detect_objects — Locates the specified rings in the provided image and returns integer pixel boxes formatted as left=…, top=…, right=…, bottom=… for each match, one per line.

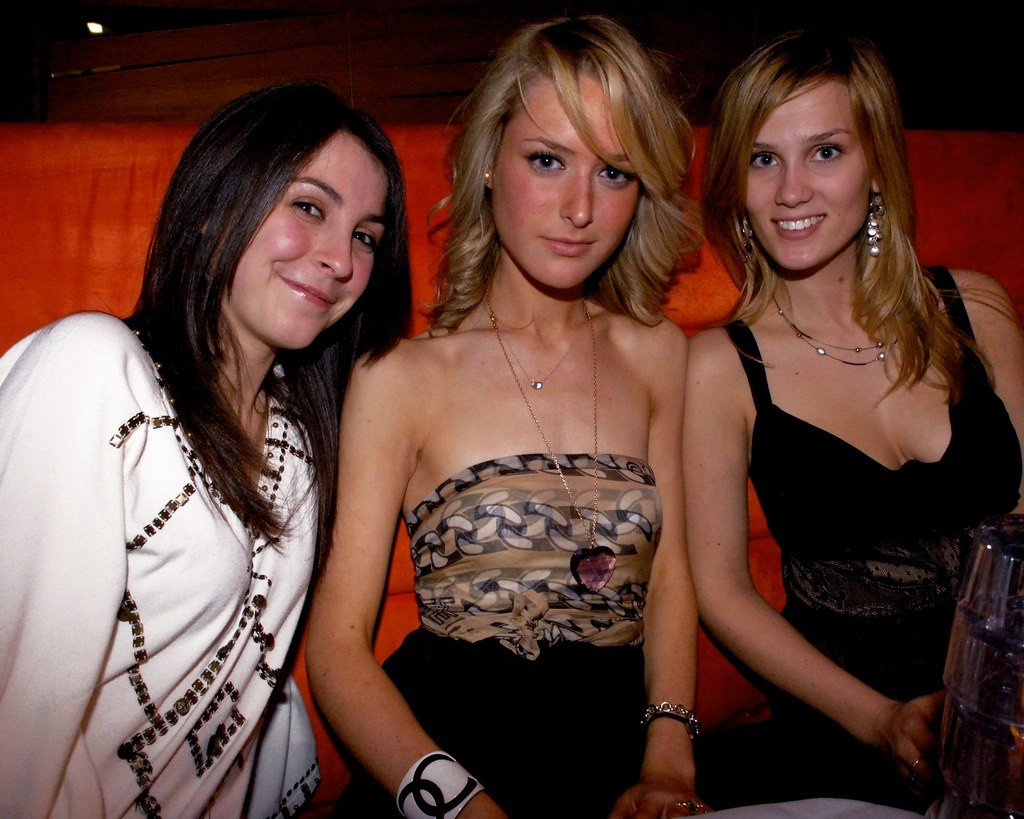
left=674, top=797, right=698, bottom=815
left=912, top=757, right=921, bottom=767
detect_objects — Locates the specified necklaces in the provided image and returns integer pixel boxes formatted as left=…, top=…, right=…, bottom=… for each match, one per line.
left=491, top=304, right=587, bottom=391
left=480, top=291, right=617, bottom=591
left=771, top=290, right=897, bottom=366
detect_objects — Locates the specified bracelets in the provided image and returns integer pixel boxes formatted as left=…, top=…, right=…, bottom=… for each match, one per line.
left=641, top=702, right=699, bottom=740
left=396, top=751, right=484, bottom=819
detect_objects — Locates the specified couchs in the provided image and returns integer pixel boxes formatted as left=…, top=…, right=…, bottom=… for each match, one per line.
left=3, top=117, right=1024, bottom=819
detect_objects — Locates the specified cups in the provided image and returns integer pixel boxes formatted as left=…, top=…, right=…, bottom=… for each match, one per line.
left=938, top=513, right=1024, bottom=819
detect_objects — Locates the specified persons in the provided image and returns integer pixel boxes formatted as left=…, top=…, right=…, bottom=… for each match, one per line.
left=0, top=83, right=411, bottom=819
left=309, top=13, right=690, bottom=819
left=684, top=27, right=1023, bottom=813
